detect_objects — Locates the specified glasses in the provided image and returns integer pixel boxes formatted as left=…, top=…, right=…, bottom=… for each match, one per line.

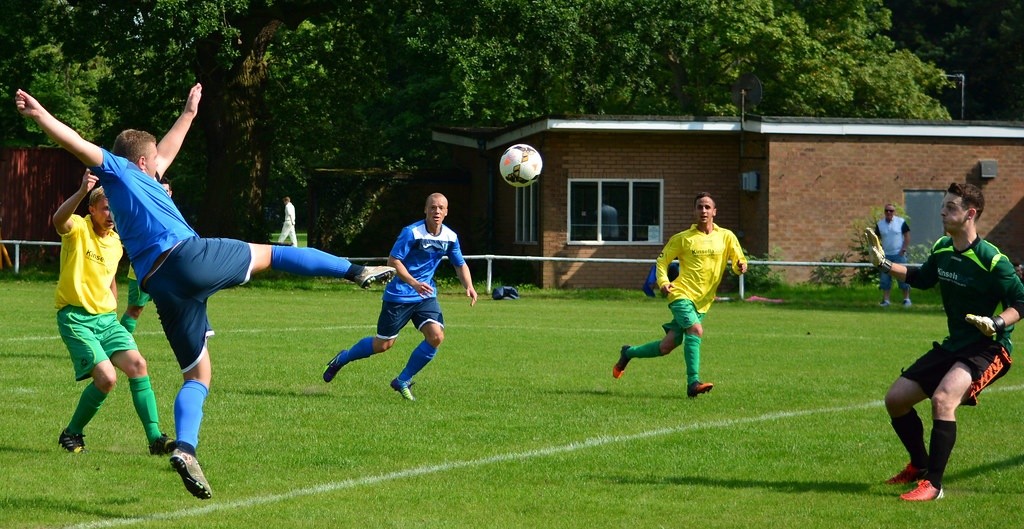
left=885, top=209, right=895, bottom=213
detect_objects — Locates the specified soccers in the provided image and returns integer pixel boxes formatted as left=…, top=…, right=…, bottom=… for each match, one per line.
left=500, top=143, right=542, bottom=187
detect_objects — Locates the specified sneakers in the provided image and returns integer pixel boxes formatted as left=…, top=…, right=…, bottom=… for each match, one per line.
left=688, top=381, right=713, bottom=398
left=880, top=300, right=890, bottom=306
left=887, top=462, right=930, bottom=484
left=391, top=377, right=414, bottom=401
left=323, top=350, right=346, bottom=382
left=150, top=436, right=178, bottom=454
left=900, top=479, right=945, bottom=502
left=356, top=266, right=397, bottom=289
left=170, top=449, right=212, bottom=499
left=59, top=428, right=84, bottom=453
left=613, top=346, right=630, bottom=379
left=902, top=299, right=911, bottom=306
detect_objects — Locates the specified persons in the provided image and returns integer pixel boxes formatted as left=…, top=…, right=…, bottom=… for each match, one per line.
left=16, top=82, right=396, bottom=500
left=875, top=206, right=911, bottom=306
left=120, top=177, right=172, bottom=335
left=278, top=197, right=297, bottom=247
left=323, top=193, right=478, bottom=400
left=864, top=183, right=1024, bottom=501
left=53, top=169, right=177, bottom=456
left=613, top=192, right=747, bottom=397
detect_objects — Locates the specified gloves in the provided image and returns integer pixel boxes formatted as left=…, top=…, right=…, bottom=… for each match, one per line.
left=863, top=227, right=884, bottom=267
left=965, top=313, right=996, bottom=336
